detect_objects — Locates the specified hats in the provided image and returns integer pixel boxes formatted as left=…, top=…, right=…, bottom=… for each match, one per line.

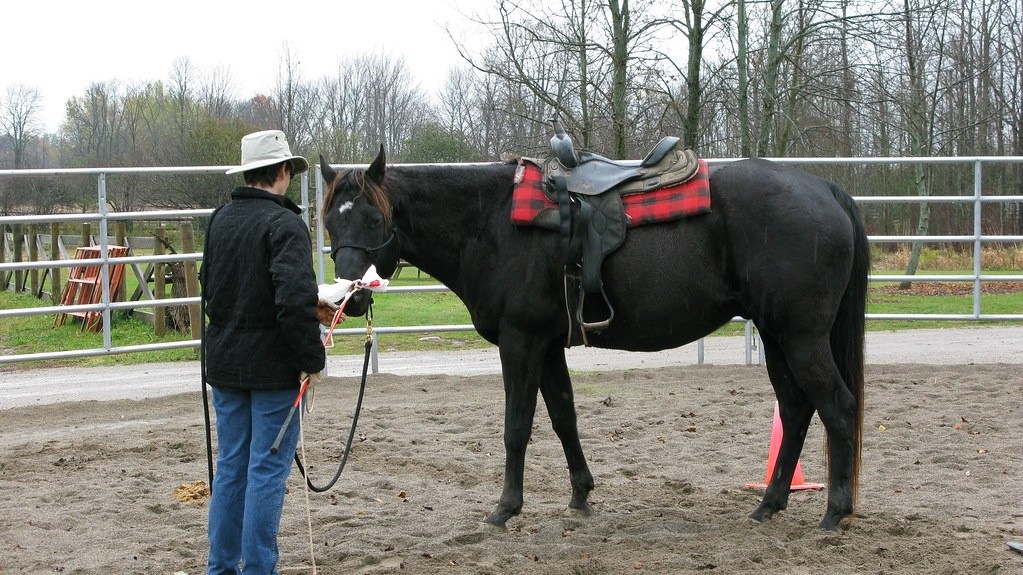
left=225, top=129, right=309, bottom=175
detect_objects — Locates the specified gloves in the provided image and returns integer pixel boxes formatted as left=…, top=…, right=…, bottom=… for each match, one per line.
left=299, top=371, right=322, bottom=389
left=316, top=297, right=346, bottom=326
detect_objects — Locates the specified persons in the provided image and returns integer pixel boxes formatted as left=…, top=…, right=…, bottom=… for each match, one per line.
left=200, top=130, right=345, bottom=575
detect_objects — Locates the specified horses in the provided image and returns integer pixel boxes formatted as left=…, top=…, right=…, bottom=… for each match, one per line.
left=319, top=143, right=871, bottom=534
